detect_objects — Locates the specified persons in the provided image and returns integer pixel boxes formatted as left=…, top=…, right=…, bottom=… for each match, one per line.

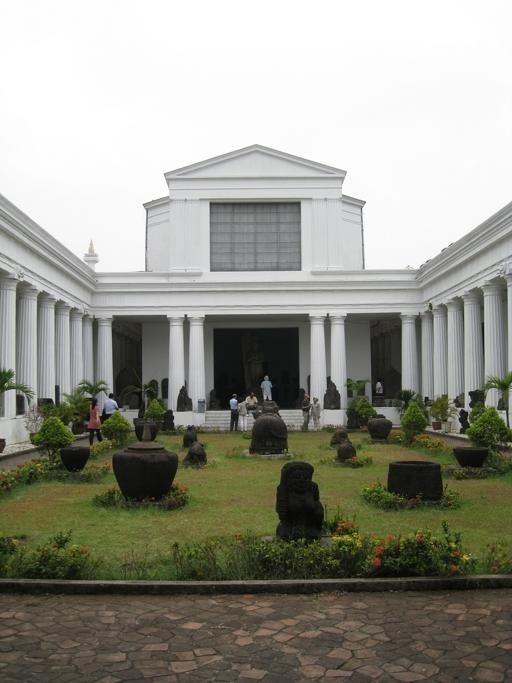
left=247, top=343, right=264, bottom=385
left=260, top=375, right=276, bottom=402
left=375, top=377, right=384, bottom=406
left=237, top=398, right=249, bottom=431
left=228, top=393, right=240, bottom=432
left=104, top=392, right=119, bottom=418
left=309, top=397, right=322, bottom=431
left=245, top=392, right=258, bottom=410
left=88, top=397, right=103, bottom=446
left=300, top=394, right=312, bottom=433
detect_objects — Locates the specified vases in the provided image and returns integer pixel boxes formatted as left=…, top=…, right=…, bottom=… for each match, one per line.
left=59, top=447, right=91, bottom=472
left=453, top=446, right=489, bottom=468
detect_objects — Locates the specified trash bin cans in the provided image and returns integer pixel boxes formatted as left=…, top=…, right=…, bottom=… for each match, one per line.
left=198, top=399, right=205, bottom=413
left=16, top=394, right=25, bottom=415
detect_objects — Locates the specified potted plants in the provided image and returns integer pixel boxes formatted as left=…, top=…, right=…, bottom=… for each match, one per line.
left=64, top=391, right=91, bottom=435
left=354, top=380, right=371, bottom=395
left=79, top=379, right=110, bottom=409
left=396, top=390, right=415, bottom=421
left=346, top=378, right=356, bottom=397
left=0, top=366, right=36, bottom=453
left=430, top=396, right=447, bottom=430
